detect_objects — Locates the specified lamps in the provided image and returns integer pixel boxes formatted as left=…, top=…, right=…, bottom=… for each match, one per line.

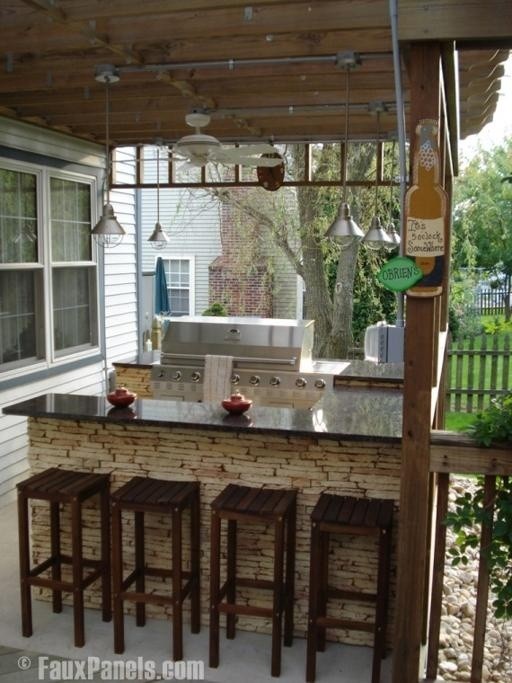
left=362, top=100, right=393, bottom=250
left=88, top=64, right=125, bottom=250
left=323, top=53, right=364, bottom=252
left=146, top=136, right=168, bottom=252
left=383, top=102, right=402, bottom=252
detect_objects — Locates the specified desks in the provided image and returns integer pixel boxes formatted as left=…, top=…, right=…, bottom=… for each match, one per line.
left=2, top=391, right=404, bottom=651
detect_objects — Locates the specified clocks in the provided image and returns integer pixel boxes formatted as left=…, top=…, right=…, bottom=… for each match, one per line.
left=256, top=149, right=287, bottom=191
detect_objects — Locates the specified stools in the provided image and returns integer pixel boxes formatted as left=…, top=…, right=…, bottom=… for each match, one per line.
left=14, top=466, right=114, bottom=648
left=305, top=491, right=396, bottom=682
left=109, top=475, right=202, bottom=661
left=208, top=481, right=299, bottom=677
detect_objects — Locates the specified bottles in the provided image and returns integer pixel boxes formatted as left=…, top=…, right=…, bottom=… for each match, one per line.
left=403, top=119, right=448, bottom=297
left=108, top=406, right=137, bottom=420
left=146, top=339, right=152, bottom=352
left=222, top=412, right=255, bottom=428
left=107, top=387, right=138, bottom=407
left=221, top=394, right=253, bottom=413
left=150, top=318, right=162, bottom=350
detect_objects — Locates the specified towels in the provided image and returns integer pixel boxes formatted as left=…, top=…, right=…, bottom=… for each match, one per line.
left=202, top=352, right=233, bottom=406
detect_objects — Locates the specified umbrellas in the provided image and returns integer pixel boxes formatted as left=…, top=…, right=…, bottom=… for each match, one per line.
left=154, top=257, right=170, bottom=317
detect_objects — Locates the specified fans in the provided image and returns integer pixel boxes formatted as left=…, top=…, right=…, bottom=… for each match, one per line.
left=108, top=109, right=284, bottom=177
